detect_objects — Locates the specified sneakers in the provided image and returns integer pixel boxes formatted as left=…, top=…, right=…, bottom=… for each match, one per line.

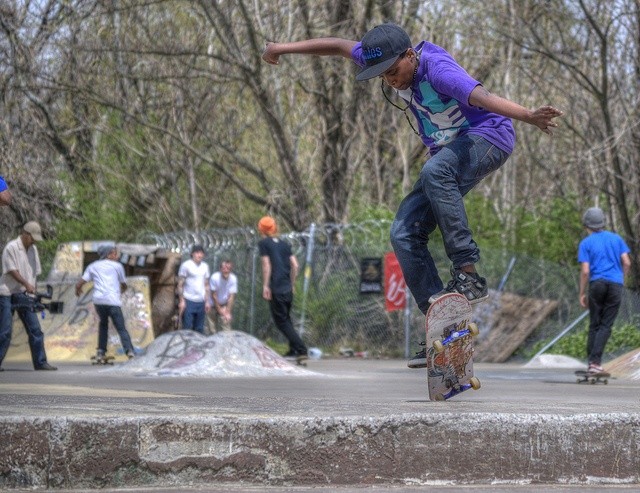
left=588, top=361, right=603, bottom=373
left=407, top=341, right=427, bottom=368
left=428, top=265, right=489, bottom=306
left=282, top=350, right=308, bottom=360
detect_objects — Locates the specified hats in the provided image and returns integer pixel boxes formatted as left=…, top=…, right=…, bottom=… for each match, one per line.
left=98, top=245, right=112, bottom=259
left=258, top=216, right=275, bottom=233
left=355, top=24, right=413, bottom=82
left=24, top=221, right=42, bottom=241
left=582, top=206, right=605, bottom=229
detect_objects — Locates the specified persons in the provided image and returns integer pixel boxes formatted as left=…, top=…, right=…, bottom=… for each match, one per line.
left=574, top=205, right=633, bottom=377
left=261, top=21, right=570, bottom=372
left=0, top=176, right=15, bottom=206
left=255, top=214, right=312, bottom=361
left=175, top=244, right=210, bottom=332
left=74, top=244, right=137, bottom=361
left=208, top=258, right=239, bottom=333
left=0, top=220, right=59, bottom=371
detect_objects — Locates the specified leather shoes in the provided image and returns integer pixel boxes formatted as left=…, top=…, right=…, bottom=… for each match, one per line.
left=35, top=363, right=57, bottom=370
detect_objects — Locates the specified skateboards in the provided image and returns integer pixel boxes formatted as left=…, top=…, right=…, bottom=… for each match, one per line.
left=574, top=370, right=611, bottom=384
left=283, top=356, right=309, bottom=367
left=425, top=294, right=480, bottom=401
left=92, top=356, right=116, bottom=365
